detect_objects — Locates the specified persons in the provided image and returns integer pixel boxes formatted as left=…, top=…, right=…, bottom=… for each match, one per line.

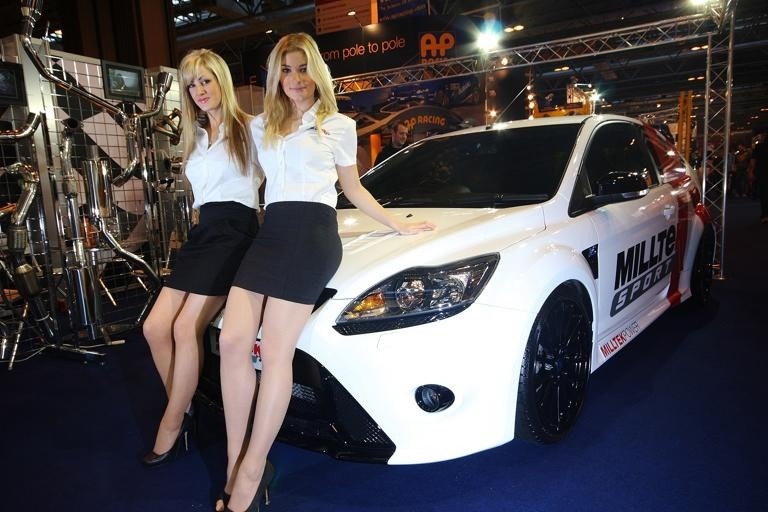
left=135, top=45, right=267, bottom=473
left=694, top=133, right=768, bottom=225
left=371, top=118, right=411, bottom=168
left=212, top=29, right=441, bottom=512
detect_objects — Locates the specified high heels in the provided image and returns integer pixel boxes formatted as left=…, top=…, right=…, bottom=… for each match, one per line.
left=223, top=458, right=276, bottom=512
left=141, top=411, right=192, bottom=466
left=214, top=488, right=231, bottom=512
left=187, top=399, right=200, bottom=436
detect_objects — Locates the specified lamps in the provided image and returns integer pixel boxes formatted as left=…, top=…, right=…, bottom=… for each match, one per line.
left=346, top=7, right=366, bottom=32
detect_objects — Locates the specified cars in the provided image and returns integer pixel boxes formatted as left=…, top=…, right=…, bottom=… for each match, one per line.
left=199, top=110, right=718, bottom=473
